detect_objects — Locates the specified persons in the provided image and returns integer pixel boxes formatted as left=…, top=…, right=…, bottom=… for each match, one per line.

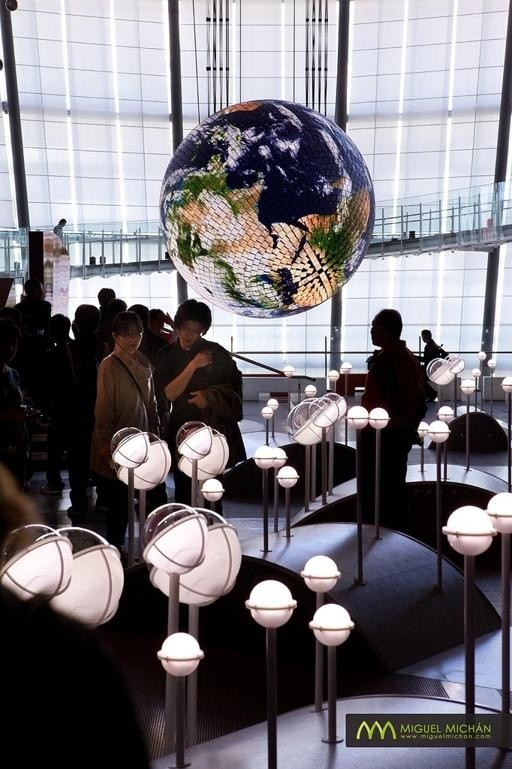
left=410, top=329, right=451, bottom=405
left=1, top=276, right=245, bottom=564
left=350, top=307, right=440, bottom=532
left=53, top=219, right=67, bottom=244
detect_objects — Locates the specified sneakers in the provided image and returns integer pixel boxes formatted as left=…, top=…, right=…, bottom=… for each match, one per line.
left=40, top=482, right=66, bottom=494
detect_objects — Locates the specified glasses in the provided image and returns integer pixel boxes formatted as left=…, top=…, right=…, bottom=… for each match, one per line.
left=117, top=331, right=144, bottom=339
left=180, top=326, right=203, bottom=335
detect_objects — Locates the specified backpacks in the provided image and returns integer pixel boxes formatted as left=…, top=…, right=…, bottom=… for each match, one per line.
left=438, top=344, right=450, bottom=360
